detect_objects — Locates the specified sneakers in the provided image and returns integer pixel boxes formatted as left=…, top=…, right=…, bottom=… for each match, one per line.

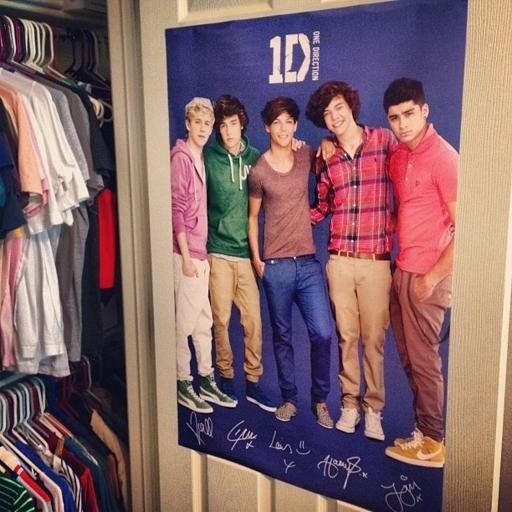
left=220, top=379, right=240, bottom=404
left=364, top=408, right=385, bottom=440
left=336, top=404, right=361, bottom=434
left=384, top=437, right=445, bottom=470
left=246, top=383, right=276, bottom=414
left=310, top=402, right=335, bottom=430
left=197, top=377, right=236, bottom=407
left=395, top=428, right=421, bottom=446
left=176, top=378, right=214, bottom=416
left=274, top=402, right=298, bottom=422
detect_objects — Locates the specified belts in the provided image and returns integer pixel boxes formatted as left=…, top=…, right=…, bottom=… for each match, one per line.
left=265, top=251, right=317, bottom=261
left=326, top=245, right=393, bottom=263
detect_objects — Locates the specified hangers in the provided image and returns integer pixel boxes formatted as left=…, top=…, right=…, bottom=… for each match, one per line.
left=0, top=13, right=116, bottom=130
left=0, top=341, right=130, bottom=482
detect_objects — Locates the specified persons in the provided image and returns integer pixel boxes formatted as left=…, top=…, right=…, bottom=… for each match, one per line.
left=383, top=75, right=458, bottom=468
left=304, top=79, right=401, bottom=443
left=202, top=93, right=307, bottom=415
left=246, top=96, right=334, bottom=430
left=170, top=95, right=237, bottom=414
